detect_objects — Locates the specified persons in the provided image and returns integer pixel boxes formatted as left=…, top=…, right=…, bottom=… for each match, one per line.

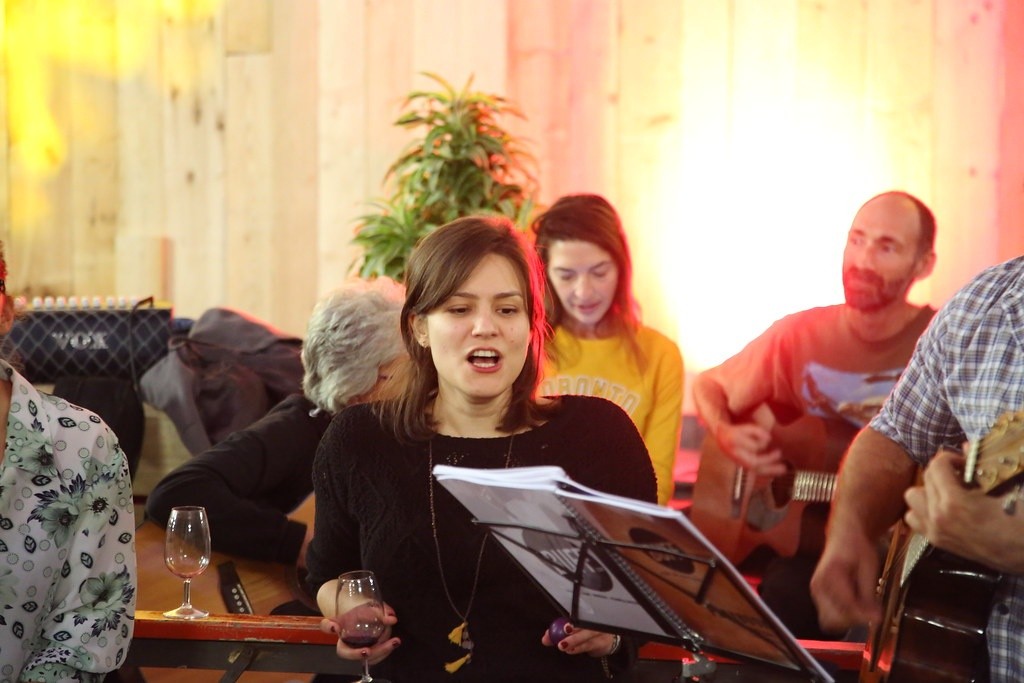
left=0, top=239, right=138, bottom=683
left=531, top=194, right=686, bottom=506
left=304, top=216, right=659, bottom=683
left=808, top=254, right=1024, bottom=683
left=145, top=275, right=421, bottom=569
left=691, top=189, right=945, bottom=644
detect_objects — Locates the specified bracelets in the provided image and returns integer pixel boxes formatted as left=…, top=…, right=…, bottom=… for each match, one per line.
left=600, top=634, right=622, bottom=680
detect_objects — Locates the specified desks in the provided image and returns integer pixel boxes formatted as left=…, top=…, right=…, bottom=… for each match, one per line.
left=126, top=520, right=365, bottom=683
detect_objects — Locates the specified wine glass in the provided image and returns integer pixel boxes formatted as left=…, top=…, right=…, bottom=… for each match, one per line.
left=162, top=505, right=212, bottom=620
left=335, top=569, right=387, bottom=683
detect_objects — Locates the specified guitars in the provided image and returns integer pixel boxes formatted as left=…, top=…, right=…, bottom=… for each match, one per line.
left=684, top=399, right=857, bottom=570
left=858, top=404, right=1024, bottom=683
